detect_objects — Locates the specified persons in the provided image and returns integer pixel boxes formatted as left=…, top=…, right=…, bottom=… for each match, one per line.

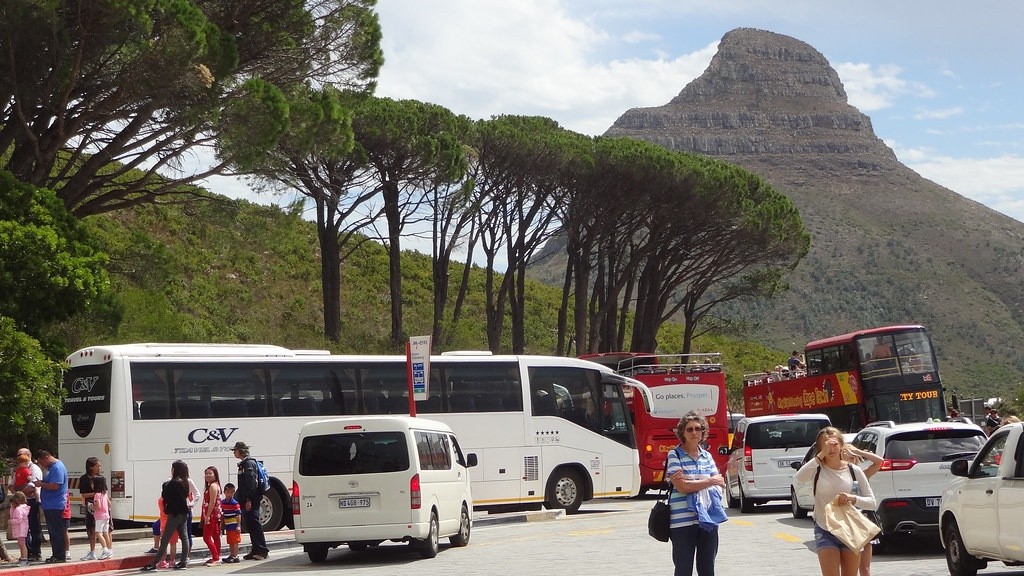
left=7, top=449, right=42, bottom=566
left=79, top=457, right=114, bottom=560
left=859, top=335, right=922, bottom=376
left=34, top=449, right=72, bottom=563
left=762, top=349, right=805, bottom=383
left=692, top=358, right=711, bottom=371
left=156, top=482, right=178, bottom=568
left=140, top=460, right=190, bottom=571
left=221, top=483, right=242, bottom=563
left=984, top=405, right=1002, bottom=437
left=144, top=478, right=200, bottom=562
left=201, top=466, right=223, bottom=567
left=231, top=441, right=269, bottom=560
left=667, top=409, right=727, bottom=576
left=841, top=443, right=883, bottom=576
left=0, top=486, right=14, bottom=562
left=947, top=406, right=959, bottom=418
left=796, top=426, right=876, bottom=576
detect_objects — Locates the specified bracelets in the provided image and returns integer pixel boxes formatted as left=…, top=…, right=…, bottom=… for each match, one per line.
left=816, top=456, right=822, bottom=462
left=247, top=499, right=250, bottom=501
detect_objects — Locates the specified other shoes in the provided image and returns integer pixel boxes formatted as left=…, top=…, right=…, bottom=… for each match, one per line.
left=98, top=549, right=113, bottom=560
left=243, top=552, right=255, bottom=560
left=205, top=558, right=222, bottom=566
left=166, top=559, right=175, bottom=567
left=0, top=554, right=42, bottom=567
left=222, top=555, right=240, bottom=563
left=65, top=551, right=71, bottom=560
left=46, top=555, right=66, bottom=563
left=155, top=561, right=166, bottom=568
left=253, top=554, right=269, bottom=560
left=144, top=548, right=159, bottom=555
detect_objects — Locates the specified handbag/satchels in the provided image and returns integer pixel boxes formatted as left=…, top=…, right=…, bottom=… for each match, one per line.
left=648, top=502, right=670, bottom=543
left=861, top=510, right=884, bottom=541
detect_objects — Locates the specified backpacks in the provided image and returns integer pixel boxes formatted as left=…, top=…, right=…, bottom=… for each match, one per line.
left=255, top=461, right=271, bottom=491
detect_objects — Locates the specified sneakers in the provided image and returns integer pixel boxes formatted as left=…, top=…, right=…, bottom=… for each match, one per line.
left=80, top=552, right=97, bottom=560
left=140, top=563, right=157, bottom=571
left=174, top=562, right=187, bottom=570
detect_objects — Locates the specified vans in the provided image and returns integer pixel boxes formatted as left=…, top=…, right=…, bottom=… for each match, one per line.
left=291, top=415, right=479, bottom=564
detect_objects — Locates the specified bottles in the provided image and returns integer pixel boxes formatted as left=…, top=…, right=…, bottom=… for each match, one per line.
left=851, top=481, right=861, bottom=496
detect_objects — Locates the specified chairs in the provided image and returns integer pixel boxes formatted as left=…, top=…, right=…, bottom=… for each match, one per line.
left=747, top=371, right=804, bottom=387
left=534, top=389, right=558, bottom=416
left=134, top=395, right=522, bottom=419
left=625, top=366, right=721, bottom=376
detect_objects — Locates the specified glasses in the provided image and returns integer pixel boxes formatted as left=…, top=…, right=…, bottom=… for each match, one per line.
left=683, top=427, right=702, bottom=432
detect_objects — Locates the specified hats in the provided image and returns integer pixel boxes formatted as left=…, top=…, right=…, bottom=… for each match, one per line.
left=231, top=441, right=249, bottom=451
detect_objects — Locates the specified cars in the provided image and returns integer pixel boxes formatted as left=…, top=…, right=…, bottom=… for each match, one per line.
left=939, top=422, right=1024, bottom=576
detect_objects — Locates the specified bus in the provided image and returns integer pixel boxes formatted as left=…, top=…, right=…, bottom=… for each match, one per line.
left=58, top=343, right=745, bottom=537
left=741, top=324, right=948, bottom=433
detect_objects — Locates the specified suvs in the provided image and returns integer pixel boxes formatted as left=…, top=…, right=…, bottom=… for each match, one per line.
left=717, top=413, right=833, bottom=515
left=790, top=417, right=990, bottom=551
